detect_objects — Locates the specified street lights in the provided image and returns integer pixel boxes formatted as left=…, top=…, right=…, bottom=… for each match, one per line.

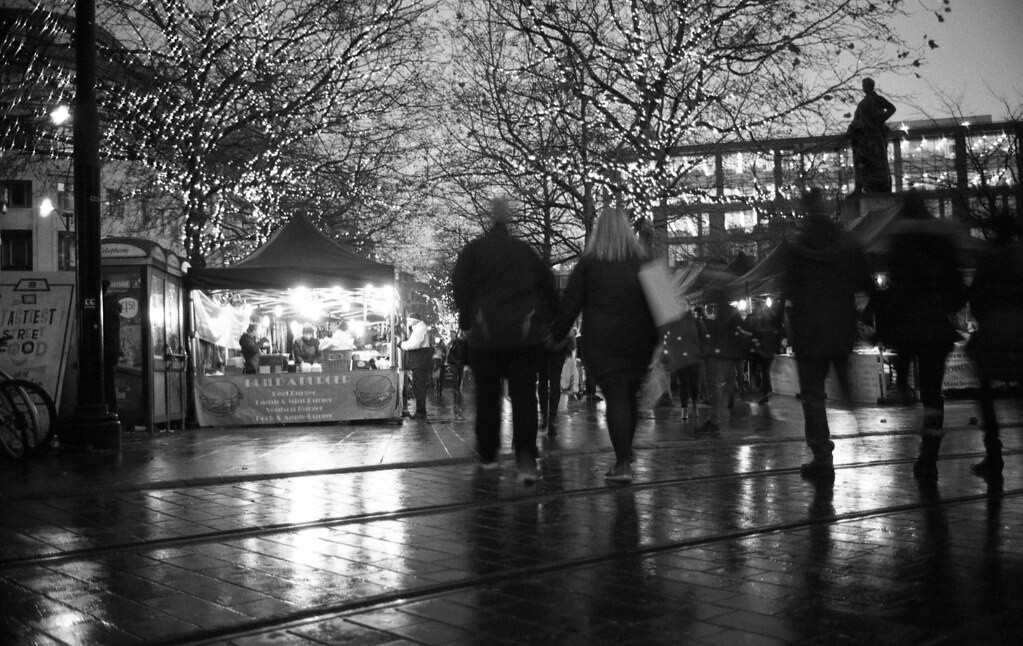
left=465, top=130, right=552, bottom=265
left=38, top=198, right=73, bottom=271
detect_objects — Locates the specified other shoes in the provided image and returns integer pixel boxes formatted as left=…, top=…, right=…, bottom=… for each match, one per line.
left=547, top=425, right=557, bottom=440
left=603, top=463, right=633, bottom=482
left=681, top=408, right=689, bottom=419
left=758, top=399, right=770, bottom=407
left=478, top=459, right=500, bottom=471
left=411, top=412, right=427, bottom=420
left=760, top=390, right=772, bottom=399
left=519, top=463, right=544, bottom=482
left=694, top=420, right=722, bottom=435
left=911, top=462, right=938, bottom=491
left=800, top=462, right=834, bottom=475
left=538, top=418, right=547, bottom=432
left=692, top=407, right=699, bottom=418
left=969, top=457, right=1006, bottom=473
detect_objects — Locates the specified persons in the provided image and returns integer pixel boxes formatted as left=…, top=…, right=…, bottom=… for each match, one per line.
left=403, top=312, right=468, bottom=419
left=240, top=311, right=320, bottom=374
left=451, top=201, right=554, bottom=484
left=966, top=211, right=1023, bottom=475
left=769, top=190, right=879, bottom=482
left=554, top=203, right=661, bottom=482
left=872, top=195, right=967, bottom=500
left=834, top=77, right=895, bottom=193
left=674, top=301, right=784, bottom=435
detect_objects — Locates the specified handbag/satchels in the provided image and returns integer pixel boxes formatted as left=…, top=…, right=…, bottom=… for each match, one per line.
left=636, top=261, right=685, bottom=329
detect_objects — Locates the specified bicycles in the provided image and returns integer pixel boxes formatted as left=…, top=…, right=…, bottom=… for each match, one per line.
left=0, top=368, right=41, bottom=462
left=0, top=379, right=57, bottom=456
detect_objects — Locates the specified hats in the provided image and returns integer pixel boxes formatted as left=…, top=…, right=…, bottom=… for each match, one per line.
left=407, top=313, right=421, bottom=321
left=302, top=326, right=315, bottom=333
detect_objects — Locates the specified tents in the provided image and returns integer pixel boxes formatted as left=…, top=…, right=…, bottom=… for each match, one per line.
left=672, top=239, right=795, bottom=300
left=182, top=212, right=395, bottom=431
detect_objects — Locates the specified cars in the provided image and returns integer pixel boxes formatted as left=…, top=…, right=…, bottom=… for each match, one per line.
left=408, top=345, right=460, bottom=395
